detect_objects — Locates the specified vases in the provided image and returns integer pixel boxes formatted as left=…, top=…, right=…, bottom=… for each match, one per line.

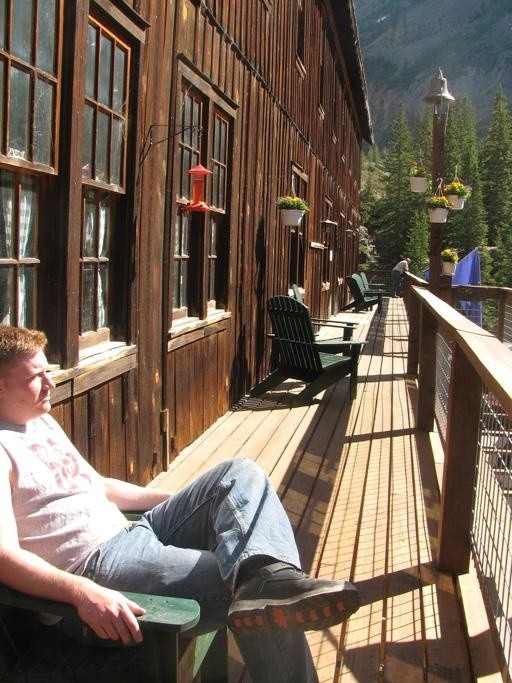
left=441, top=262, right=456, bottom=275
left=280, top=209, right=305, bottom=226
left=428, top=209, right=449, bottom=224
left=447, top=195, right=465, bottom=211
left=411, top=178, right=429, bottom=192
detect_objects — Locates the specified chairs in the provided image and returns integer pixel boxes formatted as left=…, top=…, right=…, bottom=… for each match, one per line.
left=251, top=295, right=370, bottom=406
left=293, top=284, right=359, bottom=354
left=0, top=511, right=227, bottom=683
left=345, top=272, right=392, bottom=314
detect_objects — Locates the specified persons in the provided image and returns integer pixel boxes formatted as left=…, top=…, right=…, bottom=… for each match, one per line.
left=391, top=257, right=411, bottom=298
left=0, top=323, right=366, bottom=681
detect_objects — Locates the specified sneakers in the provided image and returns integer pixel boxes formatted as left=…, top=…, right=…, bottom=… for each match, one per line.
left=226, top=561, right=362, bottom=638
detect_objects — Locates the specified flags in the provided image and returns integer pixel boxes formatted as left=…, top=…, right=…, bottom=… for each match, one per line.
left=452, top=247, right=482, bottom=329
left=424, top=268, right=430, bottom=282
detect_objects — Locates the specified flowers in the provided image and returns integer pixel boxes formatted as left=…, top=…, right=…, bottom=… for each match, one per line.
left=441, top=249, right=459, bottom=264
left=425, top=195, right=447, bottom=209
left=443, top=182, right=472, bottom=200
left=274, top=196, right=310, bottom=213
left=410, top=160, right=431, bottom=178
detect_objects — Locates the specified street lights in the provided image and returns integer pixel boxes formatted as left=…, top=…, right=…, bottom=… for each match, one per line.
left=423, top=66, right=455, bottom=289
